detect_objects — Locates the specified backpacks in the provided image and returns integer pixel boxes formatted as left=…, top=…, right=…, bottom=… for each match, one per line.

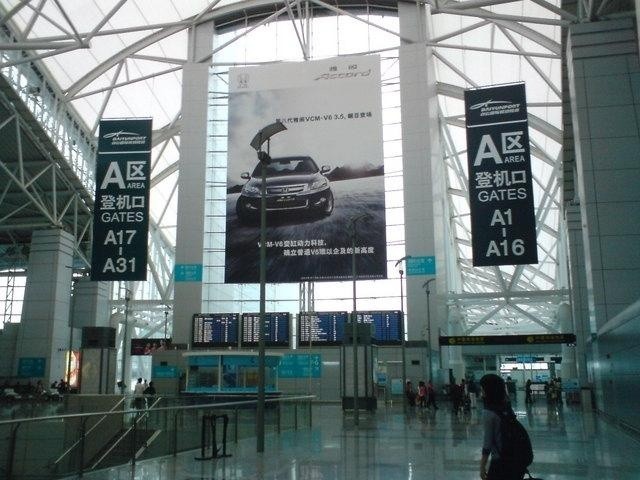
left=486, top=402, right=534, bottom=472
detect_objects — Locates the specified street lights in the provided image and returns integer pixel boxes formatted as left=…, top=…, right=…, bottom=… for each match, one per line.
left=164, top=310, right=169, bottom=339
left=118, top=286, right=132, bottom=395
left=346, top=214, right=441, bottom=434
left=64, top=266, right=87, bottom=392
left=252, top=122, right=288, bottom=461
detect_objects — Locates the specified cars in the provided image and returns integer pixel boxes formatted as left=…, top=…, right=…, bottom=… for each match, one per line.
left=237, top=154, right=335, bottom=225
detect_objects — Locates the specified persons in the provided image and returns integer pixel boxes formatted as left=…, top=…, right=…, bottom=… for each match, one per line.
left=0, top=379, right=69, bottom=398
left=404, top=409, right=567, bottom=446
left=479, top=374, right=533, bottom=480
left=134, top=378, right=155, bottom=409
left=405, top=376, right=563, bottom=409
